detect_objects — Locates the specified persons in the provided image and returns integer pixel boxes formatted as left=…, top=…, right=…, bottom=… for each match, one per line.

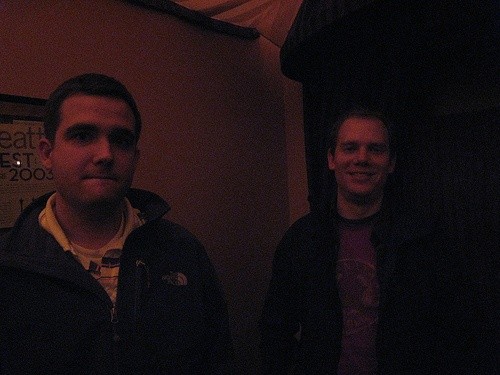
left=259, top=105, right=500, bottom=375
left=0, top=73, right=233, bottom=375
